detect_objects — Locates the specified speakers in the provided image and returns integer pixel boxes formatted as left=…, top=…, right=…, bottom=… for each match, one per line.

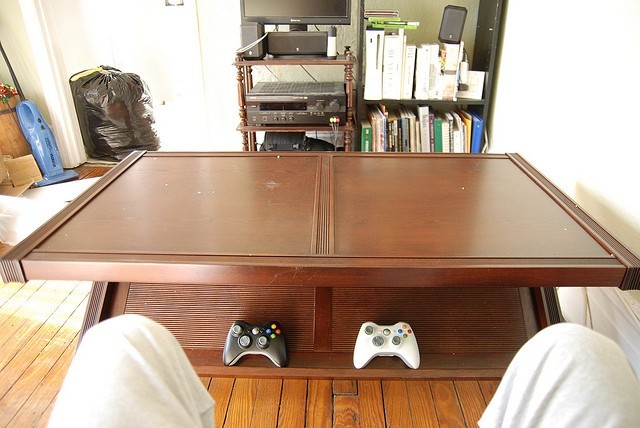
left=240, top=21, right=266, bottom=60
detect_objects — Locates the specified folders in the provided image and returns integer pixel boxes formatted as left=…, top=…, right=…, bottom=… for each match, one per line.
left=464, top=109, right=484, bottom=153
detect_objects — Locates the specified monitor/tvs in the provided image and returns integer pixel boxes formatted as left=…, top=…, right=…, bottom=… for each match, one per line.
left=240, top=0, right=351, bottom=31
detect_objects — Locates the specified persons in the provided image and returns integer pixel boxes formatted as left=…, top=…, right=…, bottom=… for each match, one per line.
left=42, top=313, right=640, bottom=428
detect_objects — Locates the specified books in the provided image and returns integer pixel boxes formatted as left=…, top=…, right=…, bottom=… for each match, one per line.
left=360, top=25, right=484, bottom=152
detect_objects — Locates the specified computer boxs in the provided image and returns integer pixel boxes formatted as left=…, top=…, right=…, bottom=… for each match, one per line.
left=266, top=30, right=327, bottom=55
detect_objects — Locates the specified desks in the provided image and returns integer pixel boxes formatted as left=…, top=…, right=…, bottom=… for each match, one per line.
left=0, top=151, right=640, bottom=379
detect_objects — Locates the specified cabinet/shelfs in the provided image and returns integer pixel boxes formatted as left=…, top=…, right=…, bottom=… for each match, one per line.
left=233, top=52, right=354, bottom=151
left=355, top=1, right=503, bottom=156
